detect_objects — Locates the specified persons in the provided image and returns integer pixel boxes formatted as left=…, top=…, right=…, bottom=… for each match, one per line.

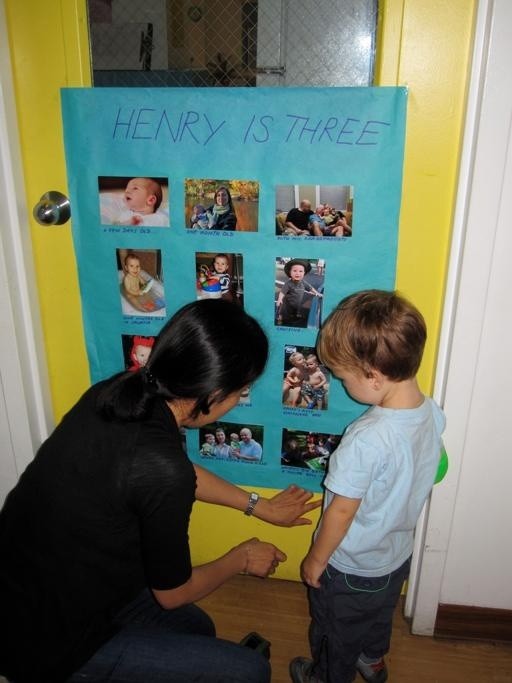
left=120, top=178, right=169, bottom=226
left=124, top=254, right=146, bottom=296
left=191, top=186, right=237, bottom=231
left=131, top=337, right=153, bottom=371
left=205, top=253, right=230, bottom=298
left=278, top=259, right=323, bottom=321
left=202, top=429, right=262, bottom=463
left=285, top=199, right=346, bottom=237
left=286, top=432, right=339, bottom=466
left=288, top=289, right=447, bottom=682
left=1, top=299, right=321, bottom=683
left=283, top=353, right=326, bottom=410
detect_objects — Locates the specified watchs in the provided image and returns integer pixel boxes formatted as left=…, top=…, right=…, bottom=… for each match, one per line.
left=245, top=492, right=259, bottom=516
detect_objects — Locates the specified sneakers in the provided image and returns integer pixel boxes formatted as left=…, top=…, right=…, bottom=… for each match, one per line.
left=290, top=656, right=355, bottom=683
left=354, top=651, right=388, bottom=683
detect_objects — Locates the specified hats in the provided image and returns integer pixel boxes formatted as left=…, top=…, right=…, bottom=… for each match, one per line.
left=281, top=259, right=315, bottom=278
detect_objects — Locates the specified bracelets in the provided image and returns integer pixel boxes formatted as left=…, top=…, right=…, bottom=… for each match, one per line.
left=236, top=547, right=249, bottom=577
